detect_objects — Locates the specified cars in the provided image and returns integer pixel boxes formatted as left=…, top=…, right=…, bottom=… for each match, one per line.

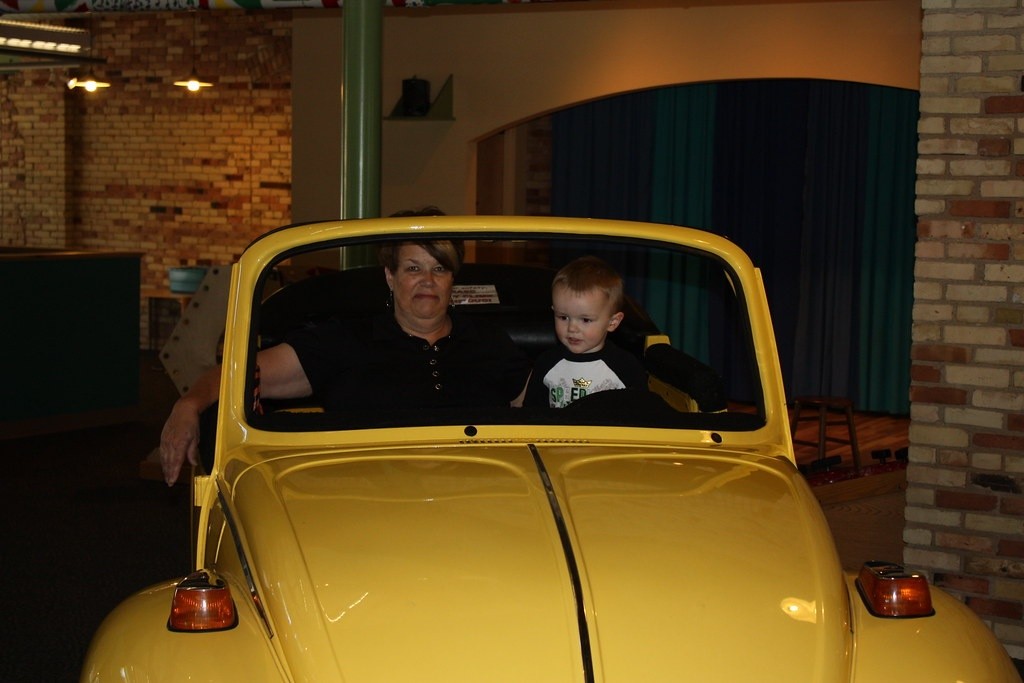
left=79, top=218, right=1024, bottom=683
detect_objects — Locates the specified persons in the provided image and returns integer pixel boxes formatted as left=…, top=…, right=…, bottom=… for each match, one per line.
left=519, top=257, right=661, bottom=397
left=160, top=212, right=533, bottom=488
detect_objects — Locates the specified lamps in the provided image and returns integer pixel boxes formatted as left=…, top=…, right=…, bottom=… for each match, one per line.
left=172, top=9, right=215, bottom=95
left=66, top=13, right=111, bottom=93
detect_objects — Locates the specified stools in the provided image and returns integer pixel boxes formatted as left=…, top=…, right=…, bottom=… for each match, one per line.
left=791, top=395, right=862, bottom=470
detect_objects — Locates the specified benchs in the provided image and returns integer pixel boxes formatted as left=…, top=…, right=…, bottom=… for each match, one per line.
left=257, top=262, right=647, bottom=432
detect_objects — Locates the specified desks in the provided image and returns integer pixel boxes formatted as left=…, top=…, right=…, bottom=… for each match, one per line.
left=142, top=287, right=194, bottom=351
